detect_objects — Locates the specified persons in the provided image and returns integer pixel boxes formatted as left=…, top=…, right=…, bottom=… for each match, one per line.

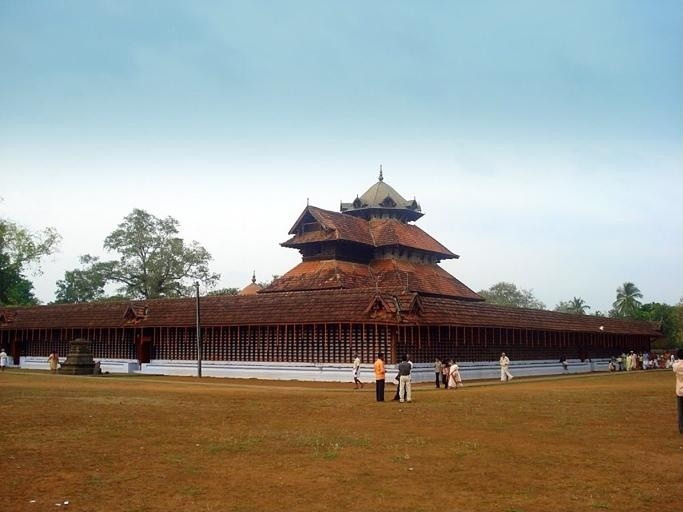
left=398, top=355, right=412, bottom=403
left=374, top=353, right=387, bottom=401
left=47, top=351, right=60, bottom=374
left=352, top=353, right=364, bottom=389
left=559, top=352, right=568, bottom=370
left=499, top=353, right=514, bottom=383
left=0, top=348, right=7, bottom=372
left=608, top=350, right=678, bottom=372
left=394, top=354, right=413, bottom=400
left=434, top=355, right=463, bottom=390
left=672, top=348, right=683, bottom=434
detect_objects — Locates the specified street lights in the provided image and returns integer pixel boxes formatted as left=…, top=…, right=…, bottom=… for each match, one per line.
left=193, top=282, right=201, bottom=378
